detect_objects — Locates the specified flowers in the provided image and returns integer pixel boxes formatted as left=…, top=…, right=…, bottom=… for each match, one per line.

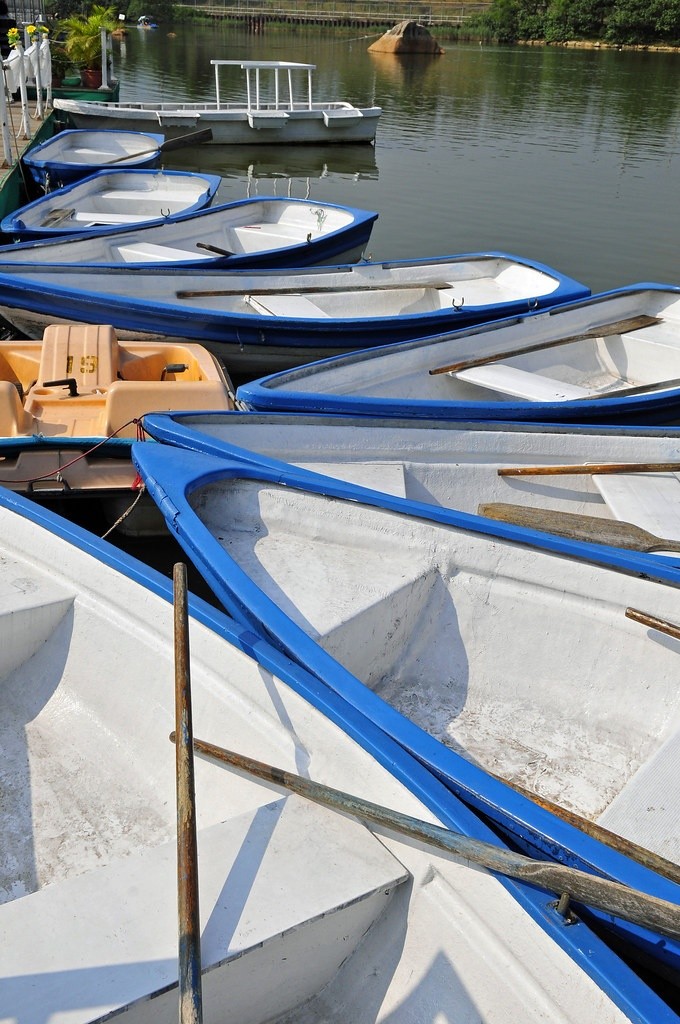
left=27, top=25, right=36, bottom=35
left=7, top=27, right=22, bottom=42
left=40, top=26, right=49, bottom=33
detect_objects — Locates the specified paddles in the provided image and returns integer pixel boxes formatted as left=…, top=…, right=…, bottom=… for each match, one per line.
left=425, top=311, right=668, bottom=380
left=99, top=125, right=216, bottom=166
left=471, top=495, right=680, bottom=571
left=567, top=372, right=680, bottom=404
left=163, top=556, right=213, bottom=1024
left=40, top=207, right=77, bottom=229
left=467, top=755, right=680, bottom=896
left=171, top=279, right=455, bottom=302
left=622, top=602, right=680, bottom=649
left=496, top=451, right=680, bottom=479
left=189, top=237, right=244, bottom=257
left=165, top=725, right=680, bottom=961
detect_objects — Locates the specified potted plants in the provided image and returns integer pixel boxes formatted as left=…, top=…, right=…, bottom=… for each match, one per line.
left=59, top=6, right=130, bottom=89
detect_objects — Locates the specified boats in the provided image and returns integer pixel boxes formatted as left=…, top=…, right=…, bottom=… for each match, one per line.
left=133, top=408, right=679, bottom=990
left=235, top=282, right=680, bottom=421
left=0, top=195, right=380, bottom=265
left=0, top=324, right=243, bottom=554
left=24, top=129, right=165, bottom=195
left=0, top=168, right=222, bottom=243
left=52, top=59, right=383, bottom=146
left=0, top=251, right=594, bottom=388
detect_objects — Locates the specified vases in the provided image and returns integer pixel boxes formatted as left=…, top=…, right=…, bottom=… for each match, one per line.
left=31, top=36, right=38, bottom=42
left=13, top=41, right=21, bottom=46
left=42, top=33, right=48, bottom=40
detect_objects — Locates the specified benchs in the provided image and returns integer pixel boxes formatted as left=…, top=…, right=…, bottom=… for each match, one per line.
left=64, top=212, right=163, bottom=227
left=92, top=188, right=200, bottom=215
left=590, top=310, right=680, bottom=392
left=444, top=362, right=601, bottom=402
left=244, top=292, right=331, bottom=320
left=62, top=145, right=150, bottom=164
left=0, top=789, right=413, bottom=1024
left=110, top=241, right=216, bottom=263
left=430, top=277, right=519, bottom=310
left=594, top=726, right=680, bottom=866
left=227, top=221, right=327, bottom=254
left=586, top=461, right=680, bottom=558
